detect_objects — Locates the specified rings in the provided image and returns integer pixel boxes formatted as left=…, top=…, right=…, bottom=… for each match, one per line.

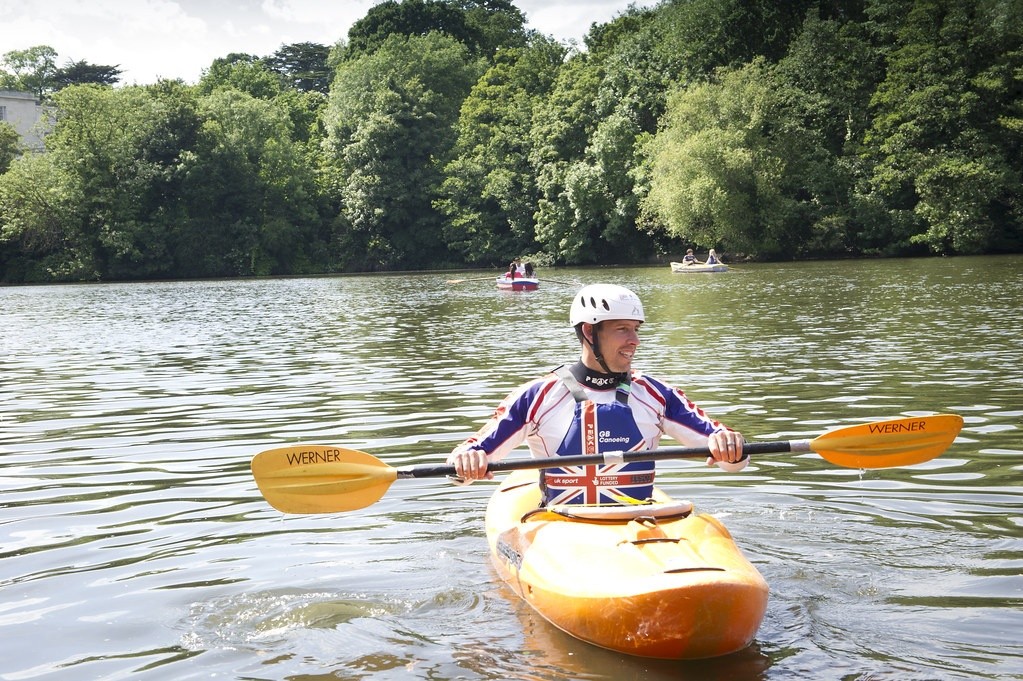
left=727, top=441, right=735, bottom=445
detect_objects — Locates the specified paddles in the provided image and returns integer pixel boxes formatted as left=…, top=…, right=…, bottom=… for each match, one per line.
left=446, top=277, right=497, bottom=284
left=252, top=413, right=963, bottom=514
left=537, top=277, right=566, bottom=284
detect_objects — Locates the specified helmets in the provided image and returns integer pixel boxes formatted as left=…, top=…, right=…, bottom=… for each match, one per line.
left=569, top=283, right=645, bottom=328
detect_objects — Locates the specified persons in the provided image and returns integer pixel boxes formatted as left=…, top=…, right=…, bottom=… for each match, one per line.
left=683, top=249, right=698, bottom=264
left=520, top=262, right=536, bottom=279
left=513, top=257, right=525, bottom=274
left=445, top=284, right=752, bottom=512
left=705, top=249, right=717, bottom=264
left=505, top=263, right=524, bottom=279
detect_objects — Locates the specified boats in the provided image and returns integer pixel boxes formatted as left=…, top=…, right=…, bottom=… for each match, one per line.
left=483, top=467, right=771, bottom=660
left=670, top=261, right=729, bottom=272
left=496, top=274, right=540, bottom=292
